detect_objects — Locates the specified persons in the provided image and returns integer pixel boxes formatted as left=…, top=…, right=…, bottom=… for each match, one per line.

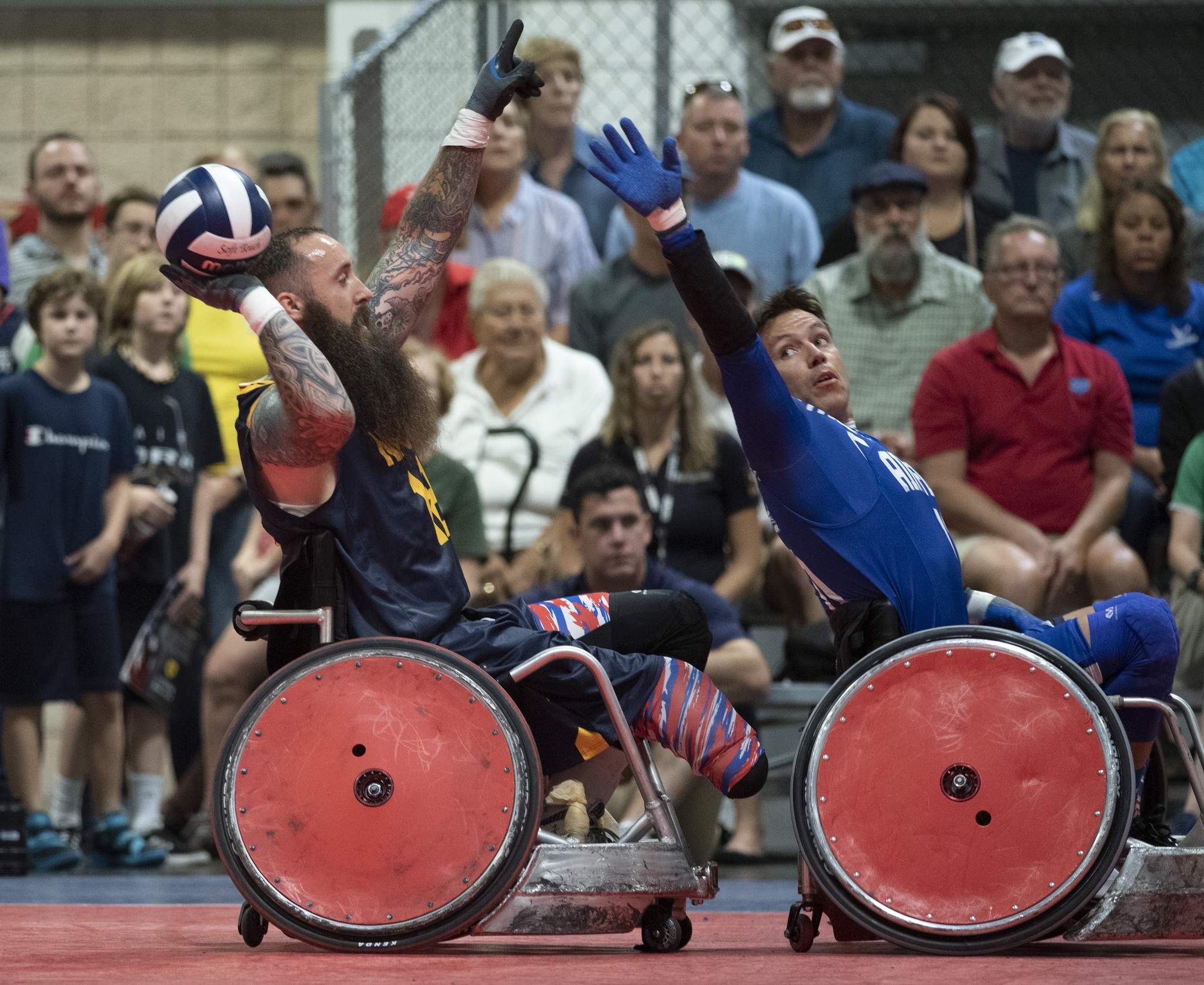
left=0, top=0, right=1204, bottom=874
left=575, top=116, right=1199, bottom=866
left=784, top=154, right=999, bottom=544
left=901, top=206, right=1151, bottom=612
left=152, top=20, right=766, bottom=800
left=522, top=317, right=762, bottom=622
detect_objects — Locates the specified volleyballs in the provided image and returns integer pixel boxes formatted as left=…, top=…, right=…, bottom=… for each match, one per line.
left=155, top=163, right=273, bottom=279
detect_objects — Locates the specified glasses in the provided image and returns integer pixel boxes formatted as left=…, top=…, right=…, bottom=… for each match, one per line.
left=858, top=191, right=923, bottom=212
left=998, top=259, right=1059, bottom=285
left=684, top=81, right=744, bottom=101
left=788, top=50, right=832, bottom=63
left=1017, top=67, right=1065, bottom=81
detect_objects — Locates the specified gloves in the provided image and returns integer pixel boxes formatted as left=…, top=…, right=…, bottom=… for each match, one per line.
left=159, top=263, right=264, bottom=314
left=466, top=19, right=545, bottom=122
left=588, top=117, right=688, bottom=232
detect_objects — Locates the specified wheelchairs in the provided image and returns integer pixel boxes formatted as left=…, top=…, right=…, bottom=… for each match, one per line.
left=208, top=599, right=717, bottom=954
left=784, top=622, right=1203, bottom=958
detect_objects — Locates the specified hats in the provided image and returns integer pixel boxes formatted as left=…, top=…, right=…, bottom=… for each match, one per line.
left=768, top=5, right=843, bottom=54
left=849, top=162, right=929, bottom=197
left=997, top=31, right=1079, bottom=72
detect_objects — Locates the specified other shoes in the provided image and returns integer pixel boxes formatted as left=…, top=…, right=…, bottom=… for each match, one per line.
left=166, top=814, right=220, bottom=863
left=1132, top=819, right=1178, bottom=847
left=25, top=811, right=79, bottom=876
left=81, top=806, right=165, bottom=870
left=720, top=837, right=777, bottom=864
